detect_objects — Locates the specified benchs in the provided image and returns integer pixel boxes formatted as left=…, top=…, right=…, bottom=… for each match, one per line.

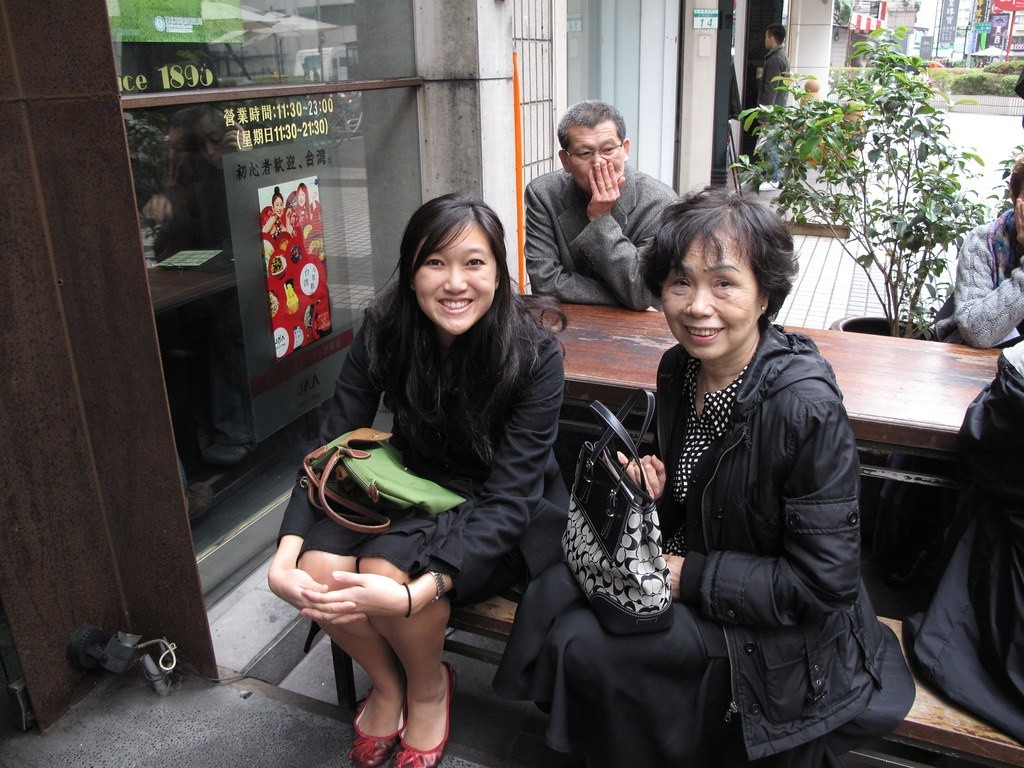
left=329, top=586, right=1024, bottom=768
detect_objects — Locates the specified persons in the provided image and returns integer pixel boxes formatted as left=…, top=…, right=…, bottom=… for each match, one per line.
left=744, top=23, right=790, bottom=187
left=493, top=188, right=915, bottom=768
left=929, top=55, right=956, bottom=89
left=144, top=99, right=254, bottom=518
left=918, top=155, right=1024, bottom=348
left=269, top=193, right=571, bottom=768
left=524, top=100, right=680, bottom=310
left=976, top=56, right=988, bottom=68
left=904, top=340, right=1024, bottom=747
left=993, top=56, right=999, bottom=63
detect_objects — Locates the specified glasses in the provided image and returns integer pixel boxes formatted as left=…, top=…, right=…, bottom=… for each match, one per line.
left=564, top=143, right=623, bottom=164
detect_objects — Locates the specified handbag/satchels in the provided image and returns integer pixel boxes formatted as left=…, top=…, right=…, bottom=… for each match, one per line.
left=298, top=427, right=467, bottom=534
left=562, top=388, right=675, bottom=639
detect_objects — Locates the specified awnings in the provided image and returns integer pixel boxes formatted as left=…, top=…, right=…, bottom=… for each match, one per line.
left=847, top=12, right=885, bottom=34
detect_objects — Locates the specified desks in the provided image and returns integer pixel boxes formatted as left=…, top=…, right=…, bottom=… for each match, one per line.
left=141, top=249, right=236, bottom=316
left=526, top=299, right=1005, bottom=487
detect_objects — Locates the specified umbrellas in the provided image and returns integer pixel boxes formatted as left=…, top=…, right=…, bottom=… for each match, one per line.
left=972, top=47, right=1014, bottom=56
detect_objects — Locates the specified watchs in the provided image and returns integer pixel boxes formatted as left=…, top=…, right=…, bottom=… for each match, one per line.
left=429, top=571, right=445, bottom=602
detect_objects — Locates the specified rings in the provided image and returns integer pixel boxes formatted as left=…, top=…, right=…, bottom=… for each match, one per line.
left=606, top=185, right=613, bottom=190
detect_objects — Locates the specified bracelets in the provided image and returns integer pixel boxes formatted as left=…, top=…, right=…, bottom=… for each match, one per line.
left=402, top=582, right=411, bottom=618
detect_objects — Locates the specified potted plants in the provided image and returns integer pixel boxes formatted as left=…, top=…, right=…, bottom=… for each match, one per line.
left=728, top=26, right=1024, bottom=345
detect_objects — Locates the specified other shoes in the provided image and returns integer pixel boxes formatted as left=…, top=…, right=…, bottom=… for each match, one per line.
left=389, top=663, right=458, bottom=768
left=350, top=681, right=405, bottom=768
